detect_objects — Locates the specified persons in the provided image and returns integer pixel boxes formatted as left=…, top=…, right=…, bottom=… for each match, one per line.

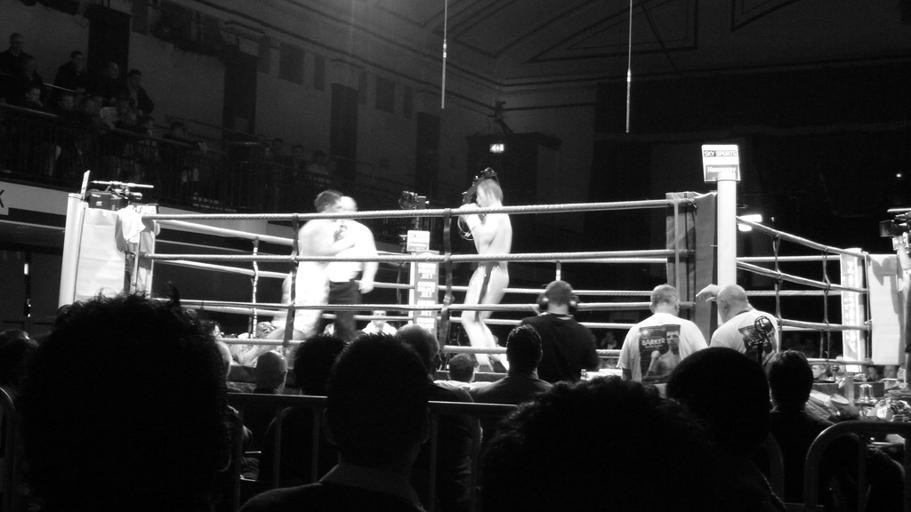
left=2, top=32, right=909, bottom=512
